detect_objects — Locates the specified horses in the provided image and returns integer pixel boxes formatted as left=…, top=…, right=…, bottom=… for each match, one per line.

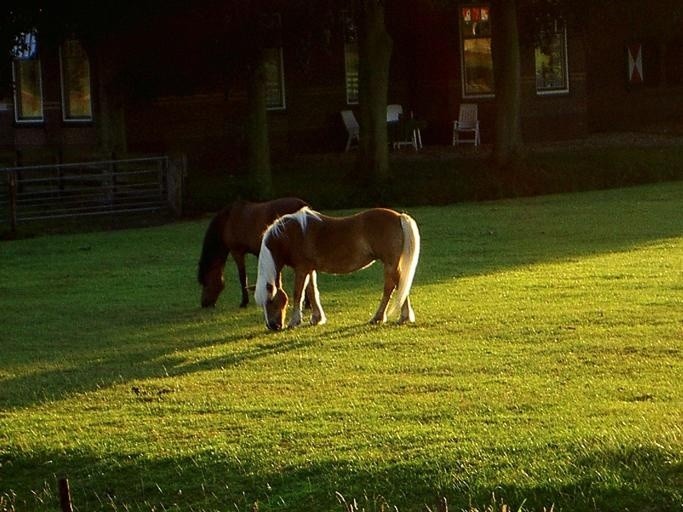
left=197, top=198, right=313, bottom=309
left=245, top=206, right=420, bottom=331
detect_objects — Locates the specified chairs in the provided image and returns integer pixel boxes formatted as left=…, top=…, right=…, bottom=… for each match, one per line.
left=452, top=103, right=481, bottom=147
left=385, top=103, right=403, bottom=121
left=340, top=110, right=360, bottom=153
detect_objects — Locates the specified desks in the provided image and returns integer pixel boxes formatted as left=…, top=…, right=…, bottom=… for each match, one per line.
left=386, top=120, right=427, bottom=151
